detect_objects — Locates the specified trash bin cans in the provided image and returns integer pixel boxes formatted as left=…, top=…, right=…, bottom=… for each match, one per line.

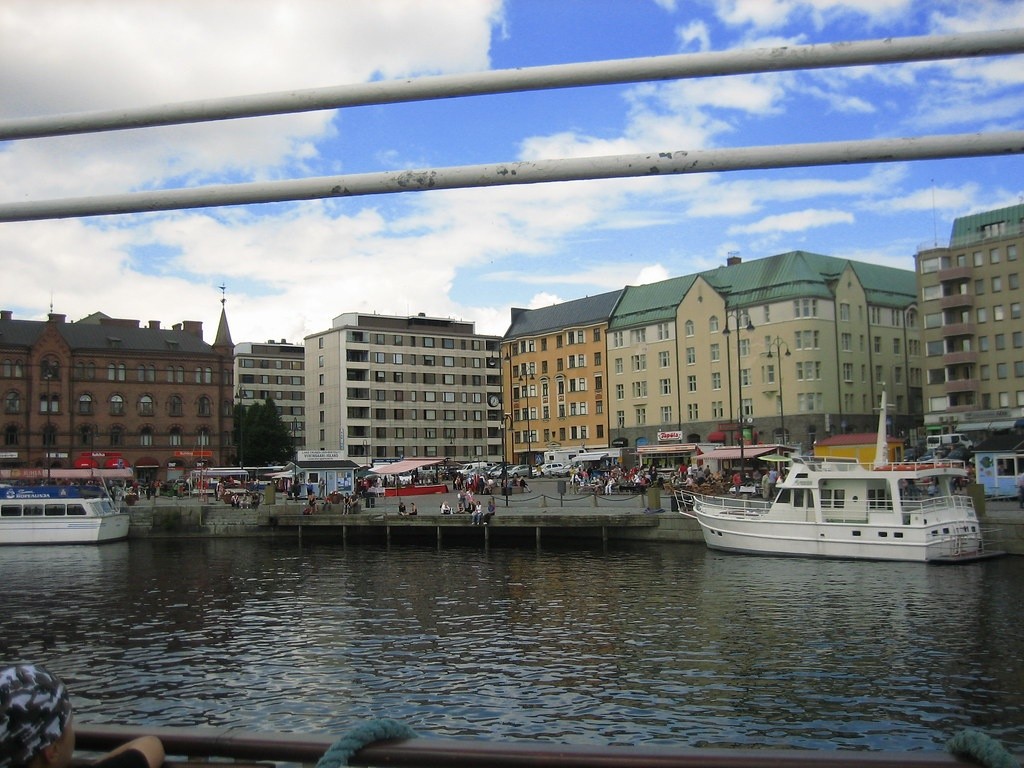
left=557, top=480, right=566, bottom=493
left=365, top=492, right=375, bottom=508
left=671, top=495, right=693, bottom=511
left=264, top=485, right=275, bottom=504
left=502, top=487, right=512, bottom=496
left=177, top=483, right=184, bottom=500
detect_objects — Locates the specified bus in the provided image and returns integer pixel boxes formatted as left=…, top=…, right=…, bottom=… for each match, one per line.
left=191, top=470, right=249, bottom=495
left=209, top=466, right=287, bottom=490
left=0, top=467, right=140, bottom=502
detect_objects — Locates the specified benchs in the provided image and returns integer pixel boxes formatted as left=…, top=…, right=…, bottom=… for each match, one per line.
left=575, top=483, right=646, bottom=495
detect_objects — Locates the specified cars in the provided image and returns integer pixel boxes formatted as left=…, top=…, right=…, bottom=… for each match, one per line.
left=902, top=448, right=918, bottom=462
left=457, top=462, right=577, bottom=479
left=917, top=445, right=969, bottom=467
left=143, top=478, right=191, bottom=494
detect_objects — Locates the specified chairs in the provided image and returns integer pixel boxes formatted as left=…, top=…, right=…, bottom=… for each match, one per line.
left=663, top=472, right=733, bottom=495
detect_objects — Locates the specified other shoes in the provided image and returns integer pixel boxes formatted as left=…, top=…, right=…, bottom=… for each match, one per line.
left=484, top=522, right=487, bottom=524
left=472, top=522, right=475, bottom=524
left=477, top=522, right=479, bottom=525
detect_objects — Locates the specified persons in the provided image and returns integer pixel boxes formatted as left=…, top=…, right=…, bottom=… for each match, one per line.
left=451, top=471, right=532, bottom=493
left=568, top=464, right=658, bottom=495
left=439, top=487, right=496, bottom=525
left=667, top=461, right=742, bottom=498
left=897, top=472, right=1024, bottom=509
left=750, top=468, right=788, bottom=499
left=305, top=478, right=333, bottom=513
left=409, top=503, right=417, bottom=515
left=198, top=475, right=261, bottom=511
left=343, top=490, right=359, bottom=515
left=397, top=502, right=409, bottom=516
left=13, top=478, right=163, bottom=504
left=358, top=474, right=404, bottom=494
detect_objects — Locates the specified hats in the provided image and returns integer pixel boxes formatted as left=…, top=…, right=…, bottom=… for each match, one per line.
left=0, top=664, right=71, bottom=768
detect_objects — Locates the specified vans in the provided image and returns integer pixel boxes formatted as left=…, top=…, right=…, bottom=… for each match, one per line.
left=925, top=433, right=973, bottom=454
left=417, top=464, right=446, bottom=483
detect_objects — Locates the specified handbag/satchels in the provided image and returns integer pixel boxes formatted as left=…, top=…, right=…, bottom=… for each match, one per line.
left=444, top=510, right=450, bottom=514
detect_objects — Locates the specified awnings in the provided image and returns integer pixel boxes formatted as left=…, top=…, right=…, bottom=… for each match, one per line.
left=735, top=430, right=757, bottom=441
left=691, top=447, right=775, bottom=460
left=687, top=433, right=701, bottom=443
left=955, top=419, right=1024, bottom=432
left=611, top=437, right=629, bottom=446
left=572, top=454, right=605, bottom=461
left=376, top=458, right=463, bottom=484
left=707, top=432, right=726, bottom=441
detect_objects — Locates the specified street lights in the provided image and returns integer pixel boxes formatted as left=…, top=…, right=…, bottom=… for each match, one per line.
left=720, top=303, right=757, bottom=485
left=766, top=334, right=792, bottom=445
left=42, top=367, right=57, bottom=485
left=289, top=417, right=302, bottom=501
left=235, top=386, right=248, bottom=486
left=489, top=337, right=511, bottom=494
left=517, top=364, right=535, bottom=480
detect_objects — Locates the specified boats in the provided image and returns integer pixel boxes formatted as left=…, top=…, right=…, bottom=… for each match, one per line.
left=691, top=454, right=1007, bottom=564
left=0, top=485, right=132, bottom=546
